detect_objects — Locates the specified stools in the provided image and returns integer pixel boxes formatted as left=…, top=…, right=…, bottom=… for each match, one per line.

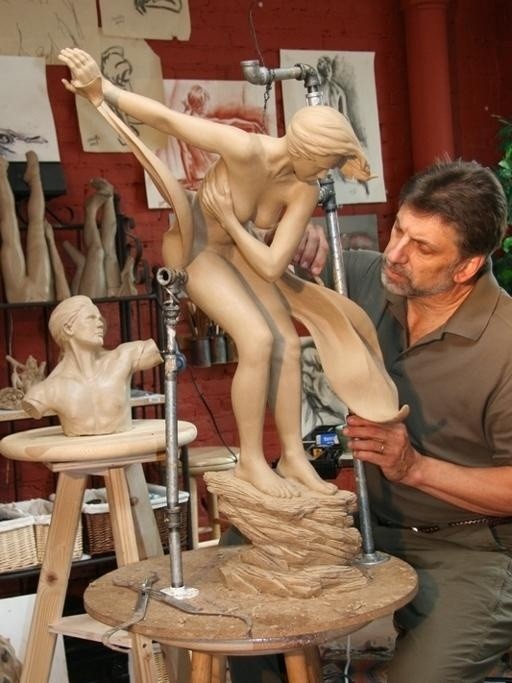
left=153, top=445, right=241, bottom=549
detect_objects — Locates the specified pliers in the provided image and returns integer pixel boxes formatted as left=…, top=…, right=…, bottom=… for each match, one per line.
left=102, top=570, right=253, bottom=653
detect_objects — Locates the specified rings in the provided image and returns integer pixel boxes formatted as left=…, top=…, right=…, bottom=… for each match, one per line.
left=380, top=443, right=385, bottom=454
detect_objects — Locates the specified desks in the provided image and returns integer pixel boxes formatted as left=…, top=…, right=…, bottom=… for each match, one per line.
left=84, top=544, right=419, bottom=683
left=0, top=419, right=198, bottom=683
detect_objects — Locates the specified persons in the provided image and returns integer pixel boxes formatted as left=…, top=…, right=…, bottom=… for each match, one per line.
left=78, top=172, right=128, bottom=300
left=310, top=50, right=350, bottom=123
left=268, top=157, right=512, bottom=682
left=2, top=151, right=57, bottom=305
left=20, top=293, right=166, bottom=437
left=175, top=75, right=273, bottom=194
left=57, top=44, right=370, bottom=505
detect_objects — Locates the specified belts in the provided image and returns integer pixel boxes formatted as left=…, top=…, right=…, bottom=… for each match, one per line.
left=369, top=509, right=512, bottom=534
